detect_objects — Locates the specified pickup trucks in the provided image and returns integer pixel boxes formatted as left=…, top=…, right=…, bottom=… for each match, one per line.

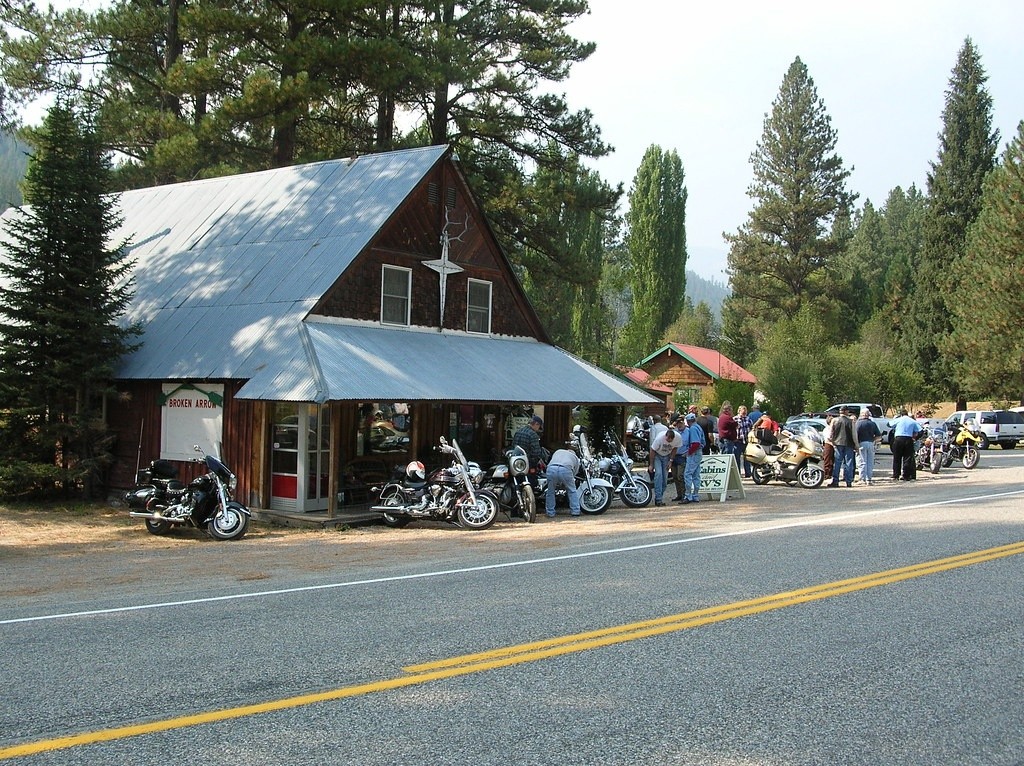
left=812, top=403, right=944, bottom=454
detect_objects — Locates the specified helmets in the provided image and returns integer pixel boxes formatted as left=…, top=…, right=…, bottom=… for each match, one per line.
left=406, top=461, right=425, bottom=480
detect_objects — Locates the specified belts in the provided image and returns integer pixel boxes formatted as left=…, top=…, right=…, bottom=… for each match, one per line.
left=551, top=464, right=564, bottom=467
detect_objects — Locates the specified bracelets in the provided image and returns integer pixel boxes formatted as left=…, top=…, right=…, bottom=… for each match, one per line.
left=670, top=459, right=673, bottom=462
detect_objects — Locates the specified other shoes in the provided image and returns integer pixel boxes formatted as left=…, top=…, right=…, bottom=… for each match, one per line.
left=678, top=498, right=690, bottom=504
left=857, top=480, right=866, bottom=485
left=511, top=511, right=523, bottom=518
left=828, top=482, right=838, bottom=487
left=655, top=500, right=665, bottom=506
left=847, top=482, right=851, bottom=487
left=672, top=497, right=684, bottom=501
left=867, top=481, right=875, bottom=485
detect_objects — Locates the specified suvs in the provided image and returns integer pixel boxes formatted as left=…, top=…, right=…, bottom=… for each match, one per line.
left=940, top=410, right=1024, bottom=449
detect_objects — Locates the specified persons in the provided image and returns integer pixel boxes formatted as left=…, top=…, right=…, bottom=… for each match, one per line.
left=822, top=406, right=926, bottom=488
left=956, top=395, right=967, bottom=411
left=627, top=400, right=780, bottom=506
left=511, top=416, right=580, bottom=518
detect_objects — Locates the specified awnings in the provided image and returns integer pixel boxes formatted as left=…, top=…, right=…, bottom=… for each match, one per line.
left=234, top=318, right=666, bottom=404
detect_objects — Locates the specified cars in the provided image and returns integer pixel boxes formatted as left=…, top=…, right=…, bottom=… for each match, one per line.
left=358, top=420, right=410, bottom=453
left=627, top=415, right=644, bottom=437
left=275, top=415, right=330, bottom=476
left=572, top=405, right=581, bottom=421
left=785, top=420, right=828, bottom=444
left=626, top=432, right=649, bottom=457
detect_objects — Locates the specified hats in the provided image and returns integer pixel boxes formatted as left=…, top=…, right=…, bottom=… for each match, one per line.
left=762, top=411, right=770, bottom=415
left=533, top=416, right=543, bottom=430
left=839, top=406, right=849, bottom=414
left=573, top=425, right=582, bottom=433
left=685, top=414, right=695, bottom=420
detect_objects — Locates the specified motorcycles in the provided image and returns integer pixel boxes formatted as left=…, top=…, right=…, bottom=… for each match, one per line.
left=744, top=421, right=825, bottom=488
left=530, top=432, right=616, bottom=514
left=573, top=424, right=652, bottom=508
left=463, top=444, right=537, bottom=524
left=125, top=444, right=251, bottom=542
left=368, top=436, right=499, bottom=530
left=915, top=423, right=953, bottom=474
left=941, top=417, right=983, bottom=469
